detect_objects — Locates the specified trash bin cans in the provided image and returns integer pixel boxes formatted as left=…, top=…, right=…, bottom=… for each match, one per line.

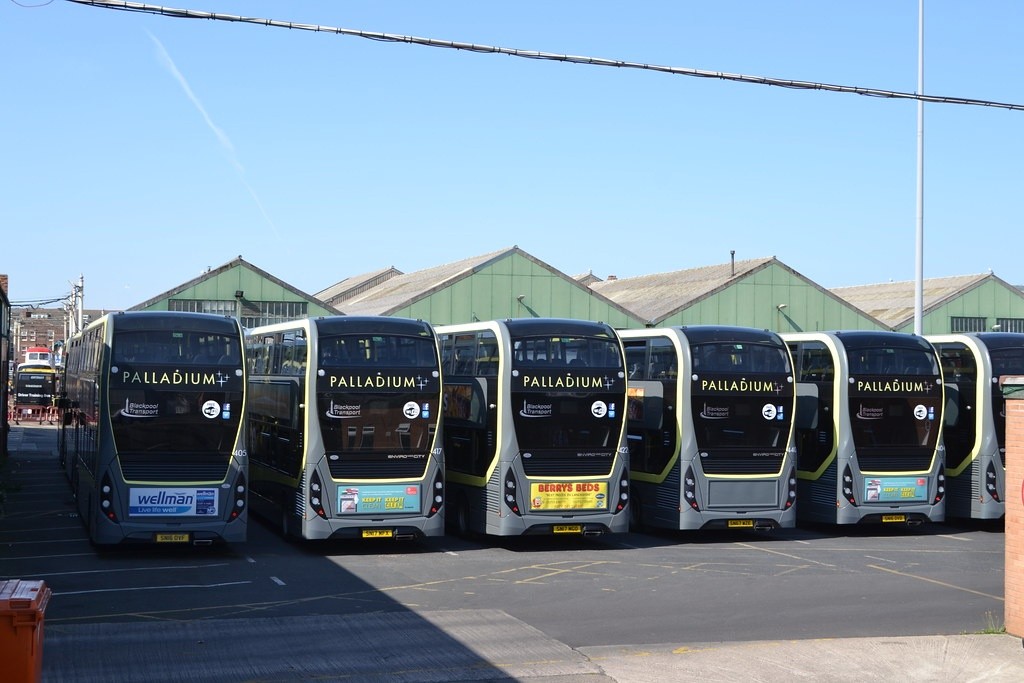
left=0, top=580, right=51, bottom=682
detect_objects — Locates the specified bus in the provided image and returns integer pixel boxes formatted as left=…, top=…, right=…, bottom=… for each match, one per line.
left=237, top=316, right=447, bottom=548
left=781, top=330, right=946, bottom=535
left=925, top=332, right=1024, bottom=521
left=431, top=320, right=631, bottom=541
left=613, top=327, right=801, bottom=539
left=55, top=311, right=249, bottom=546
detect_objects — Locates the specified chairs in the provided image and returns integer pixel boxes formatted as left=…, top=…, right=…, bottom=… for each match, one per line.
left=516, top=359, right=587, bottom=366
